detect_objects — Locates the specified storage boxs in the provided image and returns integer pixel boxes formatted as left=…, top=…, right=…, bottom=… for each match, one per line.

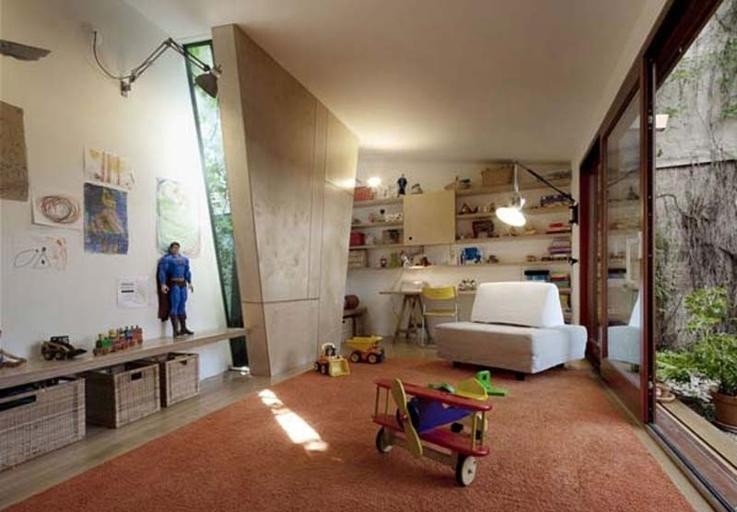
left=2, top=351, right=201, bottom=470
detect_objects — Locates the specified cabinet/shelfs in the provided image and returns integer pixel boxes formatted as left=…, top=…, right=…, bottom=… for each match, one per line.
left=350, top=169, right=575, bottom=271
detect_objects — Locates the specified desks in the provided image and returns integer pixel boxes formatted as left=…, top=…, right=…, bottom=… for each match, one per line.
left=378, top=289, right=476, bottom=337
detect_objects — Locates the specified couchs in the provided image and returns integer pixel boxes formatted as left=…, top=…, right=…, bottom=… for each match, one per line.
left=607, top=288, right=643, bottom=374
left=437, top=281, right=589, bottom=380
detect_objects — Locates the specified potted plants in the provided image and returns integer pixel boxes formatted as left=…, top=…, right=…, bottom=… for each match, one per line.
left=695, top=327, right=737, bottom=430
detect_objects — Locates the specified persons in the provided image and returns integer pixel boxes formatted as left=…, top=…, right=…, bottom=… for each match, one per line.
left=156, top=241, right=194, bottom=336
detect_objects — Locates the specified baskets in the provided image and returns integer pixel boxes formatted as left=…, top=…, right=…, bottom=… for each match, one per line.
left=481, top=166, right=513, bottom=187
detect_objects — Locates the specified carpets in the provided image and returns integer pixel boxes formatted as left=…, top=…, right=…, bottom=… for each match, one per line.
left=3, top=361, right=696, bottom=512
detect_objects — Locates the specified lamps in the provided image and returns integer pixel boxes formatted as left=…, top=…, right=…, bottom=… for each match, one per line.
left=494, top=159, right=577, bottom=229
left=389, top=250, right=423, bottom=293
left=120, top=36, right=221, bottom=97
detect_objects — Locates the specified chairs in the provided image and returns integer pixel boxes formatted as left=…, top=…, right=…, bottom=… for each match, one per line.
left=418, top=286, right=458, bottom=344
